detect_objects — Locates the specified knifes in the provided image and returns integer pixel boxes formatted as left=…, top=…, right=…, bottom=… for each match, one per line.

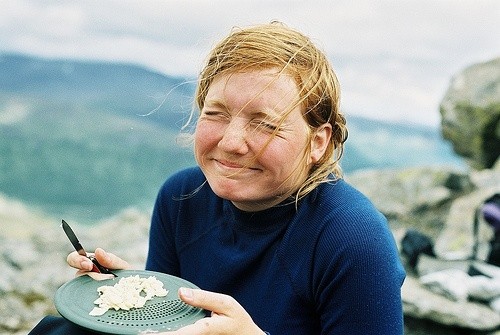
left=61, top=219, right=109, bottom=274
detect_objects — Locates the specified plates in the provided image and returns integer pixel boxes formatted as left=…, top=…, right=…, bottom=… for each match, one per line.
left=54, top=270, right=213, bottom=335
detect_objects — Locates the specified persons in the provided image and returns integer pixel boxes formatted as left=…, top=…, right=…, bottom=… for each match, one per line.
left=25, top=21, right=412, bottom=335
